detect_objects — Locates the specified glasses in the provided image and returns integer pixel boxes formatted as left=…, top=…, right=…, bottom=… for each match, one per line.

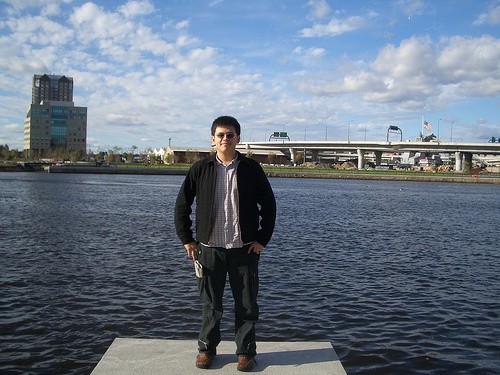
left=216, top=132, right=237, bottom=139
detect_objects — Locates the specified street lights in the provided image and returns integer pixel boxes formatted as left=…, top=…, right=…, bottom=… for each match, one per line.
left=365, top=127, right=366, bottom=141
left=283, top=123, right=288, bottom=144
left=438, top=119, right=442, bottom=145
left=325, top=126, right=328, bottom=141
left=348, top=120, right=353, bottom=144
left=305, top=126, right=310, bottom=141
left=451, top=122, right=453, bottom=143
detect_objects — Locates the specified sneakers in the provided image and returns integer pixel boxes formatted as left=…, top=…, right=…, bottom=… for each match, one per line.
left=237, top=354, right=256, bottom=372
left=196, top=349, right=215, bottom=369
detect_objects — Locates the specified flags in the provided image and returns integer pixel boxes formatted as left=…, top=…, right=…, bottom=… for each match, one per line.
left=424, top=121, right=432, bottom=130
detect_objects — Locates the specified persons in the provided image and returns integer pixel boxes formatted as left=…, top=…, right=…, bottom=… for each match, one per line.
left=175, top=116, right=276, bottom=371
